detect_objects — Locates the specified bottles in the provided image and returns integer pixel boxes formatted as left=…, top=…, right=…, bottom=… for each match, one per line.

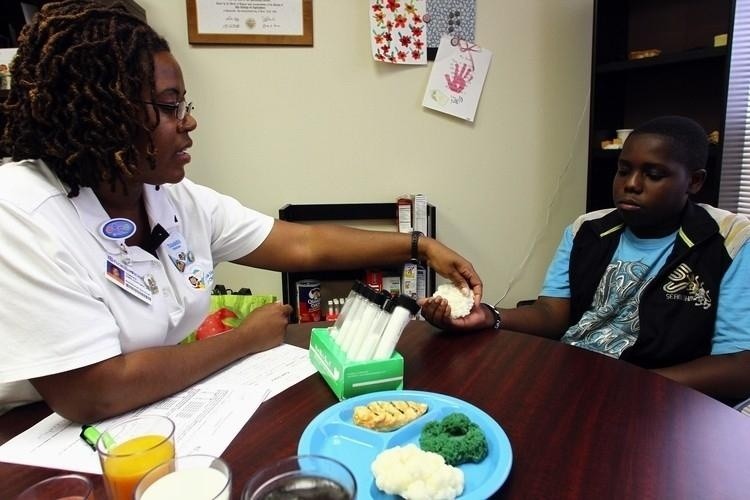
left=328, top=279, right=421, bottom=364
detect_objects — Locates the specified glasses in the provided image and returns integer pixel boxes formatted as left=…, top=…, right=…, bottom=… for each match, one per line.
left=135, top=99, right=194, bottom=121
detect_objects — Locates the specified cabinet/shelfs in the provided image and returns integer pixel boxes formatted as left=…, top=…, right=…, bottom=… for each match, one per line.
left=276, top=202, right=438, bottom=323
left=585, top=0, right=750, bottom=215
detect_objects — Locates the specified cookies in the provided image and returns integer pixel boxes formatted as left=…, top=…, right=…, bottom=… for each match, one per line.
left=352, top=398, right=488, bottom=500
left=433, top=283, right=474, bottom=320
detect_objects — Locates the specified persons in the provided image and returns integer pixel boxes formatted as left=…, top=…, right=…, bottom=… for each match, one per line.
left=421, top=112, right=749, bottom=406
left=1, top=0, right=479, bottom=425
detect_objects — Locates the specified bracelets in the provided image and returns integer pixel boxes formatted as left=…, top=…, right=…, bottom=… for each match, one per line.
left=481, top=302, right=501, bottom=329
left=411, top=231, right=420, bottom=264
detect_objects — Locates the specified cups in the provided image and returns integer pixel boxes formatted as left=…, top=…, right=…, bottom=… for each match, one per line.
left=98, top=415, right=233, bottom=500
left=240, top=454, right=359, bottom=499
left=15, top=472, right=95, bottom=500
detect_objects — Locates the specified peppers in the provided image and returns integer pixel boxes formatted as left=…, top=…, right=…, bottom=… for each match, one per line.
left=195, top=309, right=238, bottom=342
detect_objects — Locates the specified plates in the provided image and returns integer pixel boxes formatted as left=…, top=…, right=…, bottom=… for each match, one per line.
left=298, top=388, right=515, bottom=500
